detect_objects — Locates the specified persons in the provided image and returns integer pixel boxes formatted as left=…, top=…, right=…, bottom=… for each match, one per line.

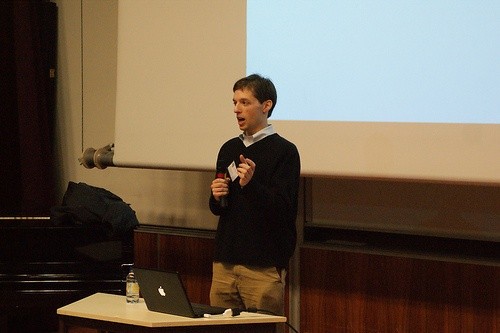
left=208, top=74, right=301, bottom=333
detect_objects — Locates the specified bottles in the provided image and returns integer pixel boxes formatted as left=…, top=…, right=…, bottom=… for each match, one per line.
left=126, top=268, right=139, bottom=304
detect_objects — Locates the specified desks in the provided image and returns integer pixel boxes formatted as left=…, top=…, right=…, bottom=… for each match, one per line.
left=56, top=291, right=287, bottom=333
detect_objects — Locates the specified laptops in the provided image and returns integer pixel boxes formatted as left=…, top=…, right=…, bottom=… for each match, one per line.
left=132, top=268, right=241, bottom=318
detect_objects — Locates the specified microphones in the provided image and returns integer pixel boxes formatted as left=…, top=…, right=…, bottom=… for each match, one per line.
left=216, top=159, right=229, bottom=208
